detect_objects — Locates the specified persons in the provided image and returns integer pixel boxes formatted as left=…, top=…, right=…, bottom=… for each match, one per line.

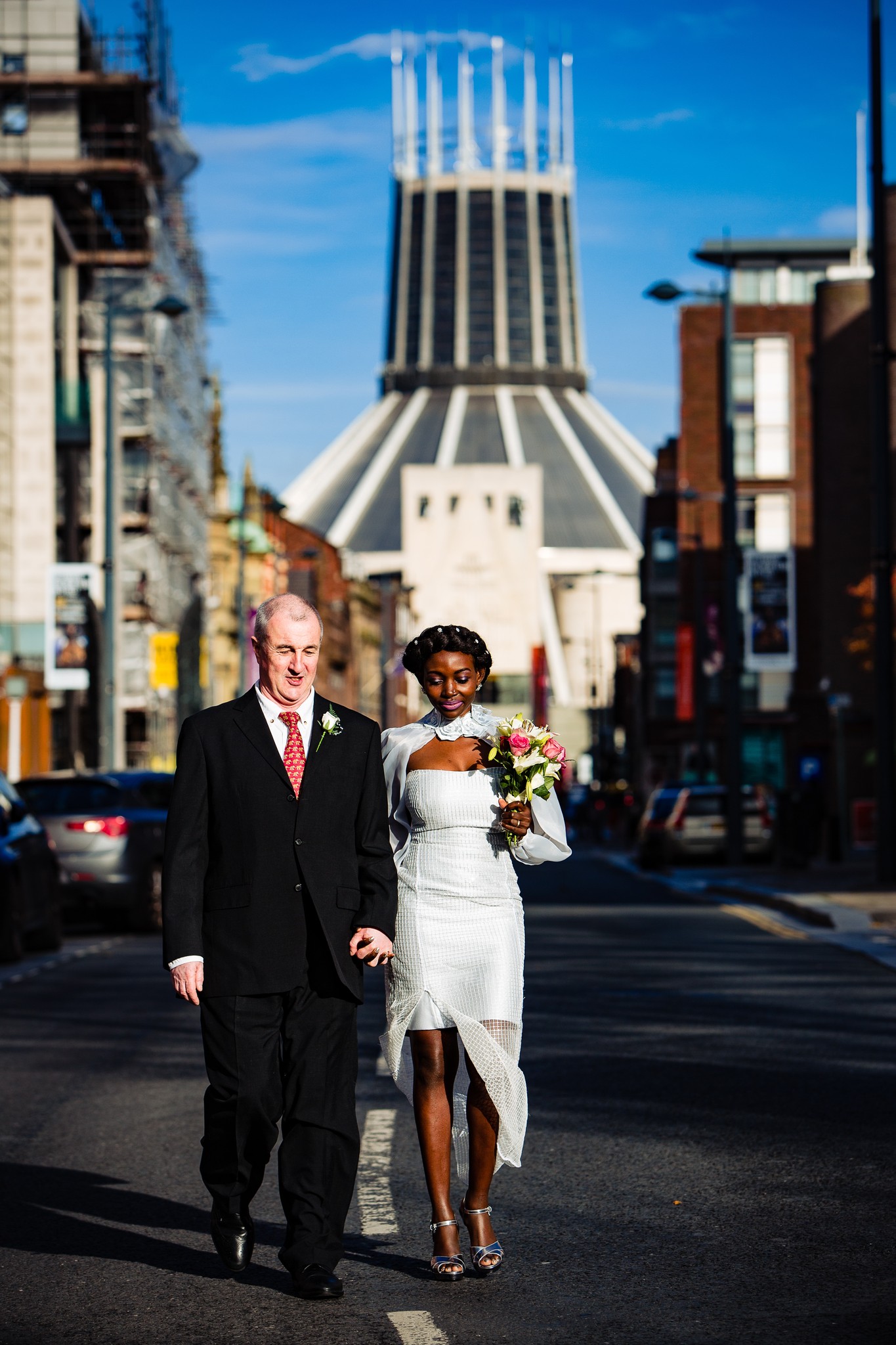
left=358, top=625, right=575, bottom=1278
left=161, top=595, right=399, bottom=1301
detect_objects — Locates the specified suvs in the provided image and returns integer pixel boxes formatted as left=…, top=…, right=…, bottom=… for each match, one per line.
left=1, top=767, right=174, bottom=965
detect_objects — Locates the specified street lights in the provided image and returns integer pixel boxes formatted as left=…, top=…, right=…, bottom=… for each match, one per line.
left=98, top=296, right=194, bottom=769
left=643, top=236, right=755, bottom=867
left=381, top=583, right=416, bottom=732
left=238, top=489, right=286, bottom=700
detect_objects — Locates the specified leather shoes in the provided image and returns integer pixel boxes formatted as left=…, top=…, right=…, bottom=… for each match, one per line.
left=292, top=1263, right=344, bottom=1299
left=209, top=1198, right=254, bottom=1272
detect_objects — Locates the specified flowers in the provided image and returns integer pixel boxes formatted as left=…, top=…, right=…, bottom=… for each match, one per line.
left=316, top=703, right=343, bottom=752
left=486, top=712, right=577, bottom=848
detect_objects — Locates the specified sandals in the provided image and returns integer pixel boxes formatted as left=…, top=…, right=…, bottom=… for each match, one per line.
left=459, top=1194, right=504, bottom=1274
left=429, top=1213, right=466, bottom=1281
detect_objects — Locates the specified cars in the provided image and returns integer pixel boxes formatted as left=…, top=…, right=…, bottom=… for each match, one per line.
left=576, top=785, right=778, bottom=869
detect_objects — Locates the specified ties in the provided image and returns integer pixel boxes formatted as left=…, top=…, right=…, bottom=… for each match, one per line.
left=278, top=712, right=307, bottom=805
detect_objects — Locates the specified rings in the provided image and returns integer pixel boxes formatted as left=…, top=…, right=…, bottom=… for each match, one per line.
left=386, top=955, right=388, bottom=958
left=516, top=820, right=520, bottom=827
